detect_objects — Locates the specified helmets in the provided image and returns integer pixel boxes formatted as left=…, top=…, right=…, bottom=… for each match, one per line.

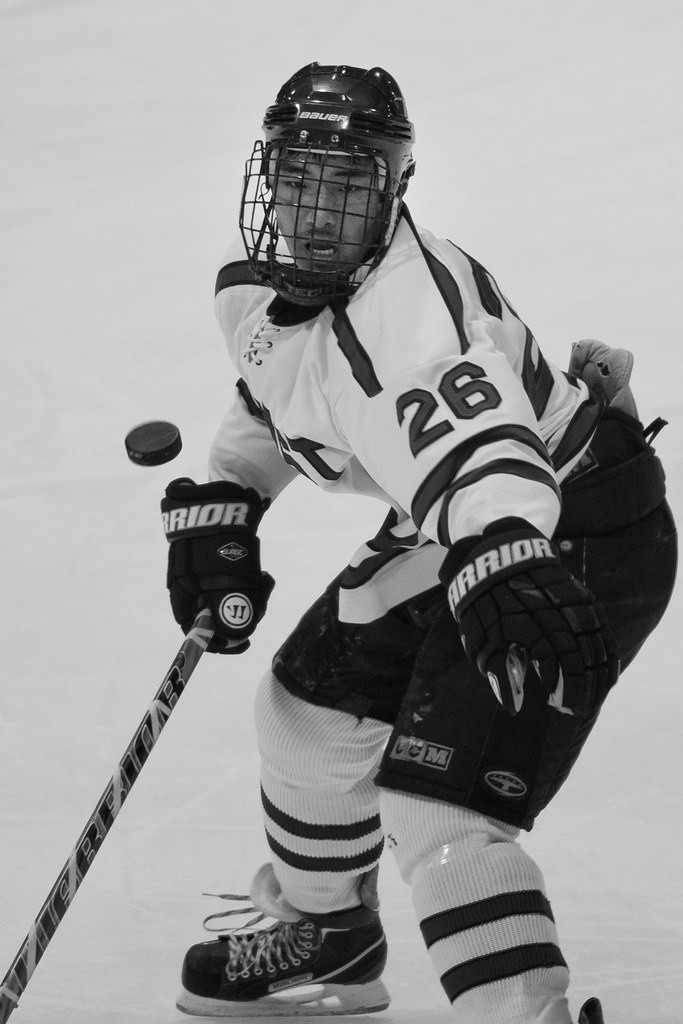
left=238, top=61, right=415, bottom=307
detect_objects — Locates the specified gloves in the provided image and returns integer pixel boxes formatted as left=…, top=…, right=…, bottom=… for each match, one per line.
left=160, top=476, right=275, bottom=655
left=438, top=514, right=619, bottom=720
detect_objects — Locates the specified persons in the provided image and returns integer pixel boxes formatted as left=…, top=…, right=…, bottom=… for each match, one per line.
left=160, top=62, right=680, bottom=1024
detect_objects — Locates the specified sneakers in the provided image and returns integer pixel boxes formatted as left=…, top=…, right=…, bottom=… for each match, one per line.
left=175, top=862, right=390, bottom=1018
